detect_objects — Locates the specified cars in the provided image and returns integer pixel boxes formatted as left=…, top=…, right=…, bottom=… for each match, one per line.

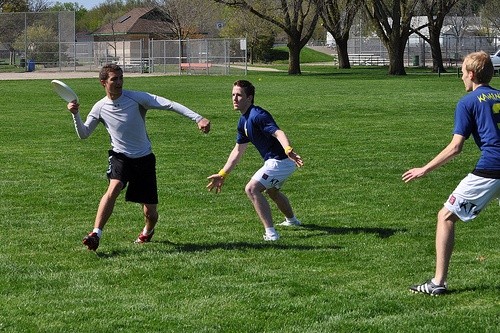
left=490, top=50, right=500, bottom=67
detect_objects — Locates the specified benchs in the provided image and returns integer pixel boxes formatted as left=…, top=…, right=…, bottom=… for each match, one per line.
left=179, top=62, right=212, bottom=74
left=331, top=53, right=387, bottom=66
left=98, top=57, right=155, bottom=72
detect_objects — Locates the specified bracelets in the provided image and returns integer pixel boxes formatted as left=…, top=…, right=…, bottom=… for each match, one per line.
left=219, top=170, right=228, bottom=178
left=284, top=146, right=291, bottom=153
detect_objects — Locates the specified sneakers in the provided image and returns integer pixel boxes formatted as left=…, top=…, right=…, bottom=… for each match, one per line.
left=133, top=230, right=154, bottom=242
left=264, top=232, right=279, bottom=241
left=83, top=233, right=100, bottom=252
left=277, top=220, right=300, bottom=225
left=410, top=281, right=448, bottom=295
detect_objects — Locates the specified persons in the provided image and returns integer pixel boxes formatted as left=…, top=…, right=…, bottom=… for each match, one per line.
left=206, top=80, right=304, bottom=241
left=402, top=51, right=500, bottom=296
left=67, top=63, right=210, bottom=250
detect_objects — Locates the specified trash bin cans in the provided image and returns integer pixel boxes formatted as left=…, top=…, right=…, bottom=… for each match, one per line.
left=29, top=59, right=35, bottom=71
left=412, top=55, right=419, bottom=66
left=20, top=59, right=25, bottom=68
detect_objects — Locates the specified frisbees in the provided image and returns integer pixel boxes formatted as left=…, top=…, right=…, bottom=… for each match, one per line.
left=51, top=79, right=79, bottom=103
